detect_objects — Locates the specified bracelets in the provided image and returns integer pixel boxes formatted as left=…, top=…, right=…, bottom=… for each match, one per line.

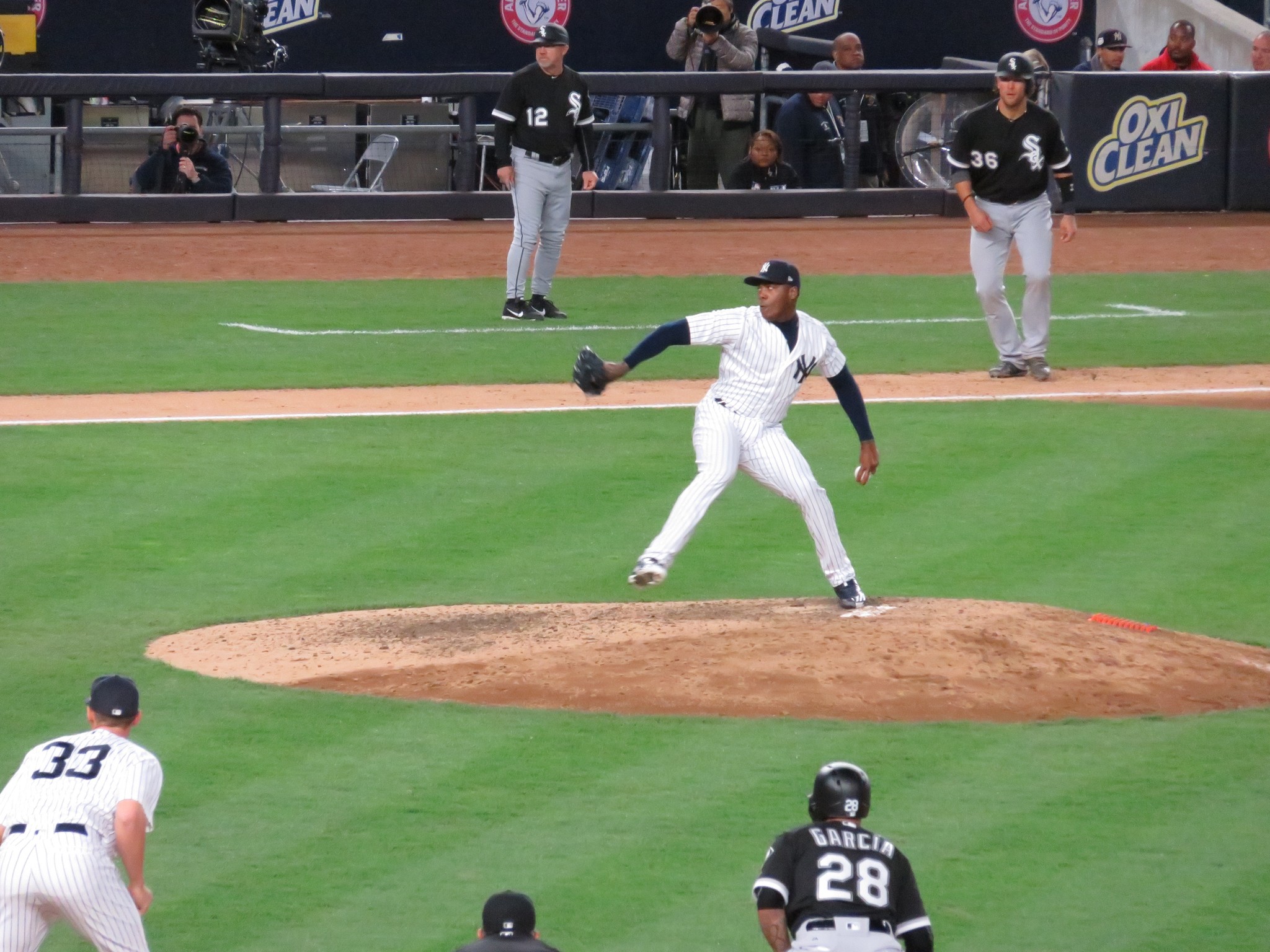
left=189, top=174, right=199, bottom=180
left=961, top=191, right=976, bottom=203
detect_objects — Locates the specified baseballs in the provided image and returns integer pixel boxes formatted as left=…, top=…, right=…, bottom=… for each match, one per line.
left=853, top=466, right=871, bottom=483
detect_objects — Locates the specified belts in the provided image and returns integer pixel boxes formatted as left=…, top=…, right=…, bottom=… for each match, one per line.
left=716, top=398, right=735, bottom=412
left=807, top=919, right=892, bottom=934
left=525, top=151, right=570, bottom=166
left=10, top=822, right=88, bottom=835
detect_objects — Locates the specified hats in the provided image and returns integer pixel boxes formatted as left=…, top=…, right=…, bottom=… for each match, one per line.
left=995, top=51, right=1035, bottom=81
left=481, top=890, right=535, bottom=934
left=813, top=61, right=838, bottom=71
left=86, top=675, right=139, bottom=717
left=530, top=24, right=569, bottom=45
left=1096, top=29, right=1134, bottom=49
left=743, top=260, right=799, bottom=286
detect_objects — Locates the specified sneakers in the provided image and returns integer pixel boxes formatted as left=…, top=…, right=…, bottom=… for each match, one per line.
left=1026, top=357, right=1051, bottom=379
left=631, top=559, right=668, bottom=586
left=834, top=579, right=864, bottom=607
left=501, top=300, right=546, bottom=321
left=990, top=361, right=1027, bottom=378
left=530, top=294, right=566, bottom=319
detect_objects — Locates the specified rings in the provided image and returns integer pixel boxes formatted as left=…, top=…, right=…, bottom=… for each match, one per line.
left=183, top=166, right=186, bottom=168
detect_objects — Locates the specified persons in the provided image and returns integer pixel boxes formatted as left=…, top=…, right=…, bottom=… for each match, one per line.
left=130, top=106, right=235, bottom=195
left=773, top=61, right=844, bottom=190
left=1252, top=30, right=1270, bottom=72
left=519, top=0, right=550, bottom=25
left=1071, top=28, right=1131, bottom=71
left=455, top=890, right=560, bottom=952
left=753, top=761, right=935, bottom=952
left=572, top=259, right=880, bottom=609
left=1137, top=20, right=1213, bottom=72
left=0, top=674, right=162, bottom=952
left=831, top=32, right=890, bottom=189
left=666, top=0, right=758, bottom=190
left=490, top=23, right=599, bottom=321
left=727, top=129, right=802, bottom=190
left=947, top=51, right=1078, bottom=382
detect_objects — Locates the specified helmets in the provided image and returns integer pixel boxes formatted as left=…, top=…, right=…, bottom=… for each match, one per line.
left=808, top=760, right=870, bottom=821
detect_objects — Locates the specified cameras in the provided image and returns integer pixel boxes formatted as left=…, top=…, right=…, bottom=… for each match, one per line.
left=694, top=4, right=723, bottom=34
left=174, top=125, right=199, bottom=146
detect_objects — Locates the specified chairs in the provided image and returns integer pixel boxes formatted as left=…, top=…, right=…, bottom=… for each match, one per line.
left=311, top=134, right=399, bottom=192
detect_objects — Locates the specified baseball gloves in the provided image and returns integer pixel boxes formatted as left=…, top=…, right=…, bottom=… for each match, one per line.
left=571, top=347, right=608, bottom=397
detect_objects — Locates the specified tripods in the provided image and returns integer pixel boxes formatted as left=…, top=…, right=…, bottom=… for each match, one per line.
left=204, top=100, right=286, bottom=194
